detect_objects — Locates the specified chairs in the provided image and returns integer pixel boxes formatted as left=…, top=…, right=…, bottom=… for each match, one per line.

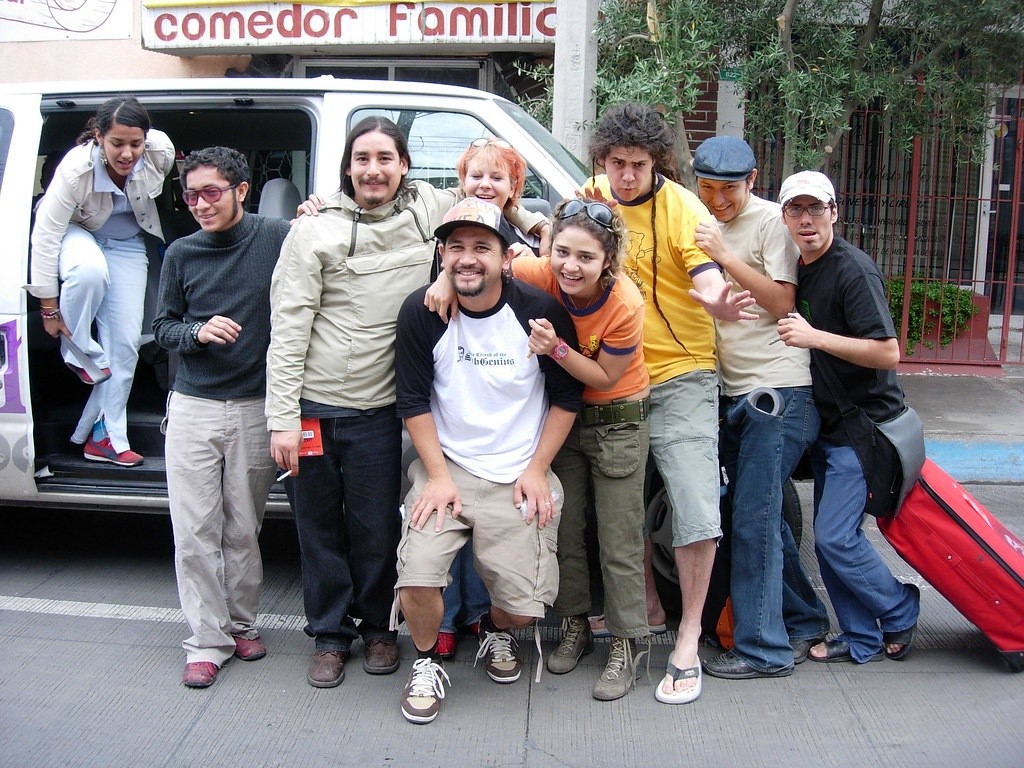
left=257, top=179, right=301, bottom=219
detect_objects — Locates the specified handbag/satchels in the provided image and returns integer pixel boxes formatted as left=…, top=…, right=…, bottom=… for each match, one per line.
left=864, top=406, right=926, bottom=519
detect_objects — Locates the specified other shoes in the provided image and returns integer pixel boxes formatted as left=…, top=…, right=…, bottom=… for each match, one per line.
left=436, top=631, right=456, bottom=657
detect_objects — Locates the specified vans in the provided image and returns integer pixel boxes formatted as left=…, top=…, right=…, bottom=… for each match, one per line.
left=0, top=74, right=596, bottom=526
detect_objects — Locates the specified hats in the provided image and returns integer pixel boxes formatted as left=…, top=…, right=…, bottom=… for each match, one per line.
left=779, top=170, right=836, bottom=208
left=693, top=136, right=756, bottom=181
left=434, top=197, right=511, bottom=247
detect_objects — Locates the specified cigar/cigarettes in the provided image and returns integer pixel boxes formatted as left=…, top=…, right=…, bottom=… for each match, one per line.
left=768, top=338, right=781, bottom=345
left=277, top=470, right=292, bottom=481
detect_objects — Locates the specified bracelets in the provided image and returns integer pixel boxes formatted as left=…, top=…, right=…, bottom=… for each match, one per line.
left=40, top=306, right=61, bottom=319
left=191, top=322, right=210, bottom=346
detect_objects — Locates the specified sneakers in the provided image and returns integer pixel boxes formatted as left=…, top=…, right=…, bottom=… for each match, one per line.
left=702, top=650, right=794, bottom=678
left=83, top=437, right=144, bottom=467
left=184, top=663, right=219, bottom=688
left=400, top=657, right=450, bottom=724
left=67, top=362, right=111, bottom=385
left=234, top=637, right=266, bottom=662
left=473, top=613, right=521, bottom=682
left=361, top=638, right=401, bottom=674
left=307, top=650, right=349, bottom=688
left=547, top=613, right=594, bottom=673
left=790, top=635, right=825, bottom=664
left=592, top=637, right=642, bottom=700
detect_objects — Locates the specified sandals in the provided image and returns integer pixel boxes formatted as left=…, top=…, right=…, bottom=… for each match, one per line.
left=807, top=639, right=886, bottom=663
left=882, top=583, right=920, bottom=660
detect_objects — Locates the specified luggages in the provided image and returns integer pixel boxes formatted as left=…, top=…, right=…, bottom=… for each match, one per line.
left=876, top=458, right=1024, bottom=674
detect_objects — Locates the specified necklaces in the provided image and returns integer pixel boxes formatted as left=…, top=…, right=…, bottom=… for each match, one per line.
left=569, top=290, right=598, bottom=310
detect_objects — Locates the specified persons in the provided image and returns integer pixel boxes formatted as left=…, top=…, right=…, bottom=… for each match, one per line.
left=693, top=134, right=816, bottom=680
left=570, top=105, right=759, bottom=704
left=151, top=147, right=324, bottom=688
left=393, top=197, right=584, bottom=724
left=423, top=199, right=650, bottom=701
left=777, top=170, right=919, bottom=661
left=431, top=139, right=539, bottom=659
left=266, top=117, right=554, bottom=686
left=22, top=97, right=176, bottom=466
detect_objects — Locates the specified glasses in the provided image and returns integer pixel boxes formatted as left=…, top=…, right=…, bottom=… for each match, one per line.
left=784, top=204, right=831, bottom=216
left=182, top=185, right=235, bottom=207
left=470, top=138, right=512, bottom=150
left=559, top=200, right=615, bottom=226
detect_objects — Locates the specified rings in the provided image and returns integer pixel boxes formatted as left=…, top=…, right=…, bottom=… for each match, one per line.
left=545, top=503, right=551, bottom=510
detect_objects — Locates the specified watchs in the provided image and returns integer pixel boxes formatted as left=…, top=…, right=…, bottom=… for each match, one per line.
left=549, top=338, right=569, bottom=360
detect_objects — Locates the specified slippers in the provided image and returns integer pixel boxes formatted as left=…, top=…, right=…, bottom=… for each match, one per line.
left=654, top=649, right=702, bottom=704
left=587, top=613, right=667, bottom=634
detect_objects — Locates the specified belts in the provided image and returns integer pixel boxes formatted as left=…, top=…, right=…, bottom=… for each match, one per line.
left=577, top=398, right=649, bottom=428
